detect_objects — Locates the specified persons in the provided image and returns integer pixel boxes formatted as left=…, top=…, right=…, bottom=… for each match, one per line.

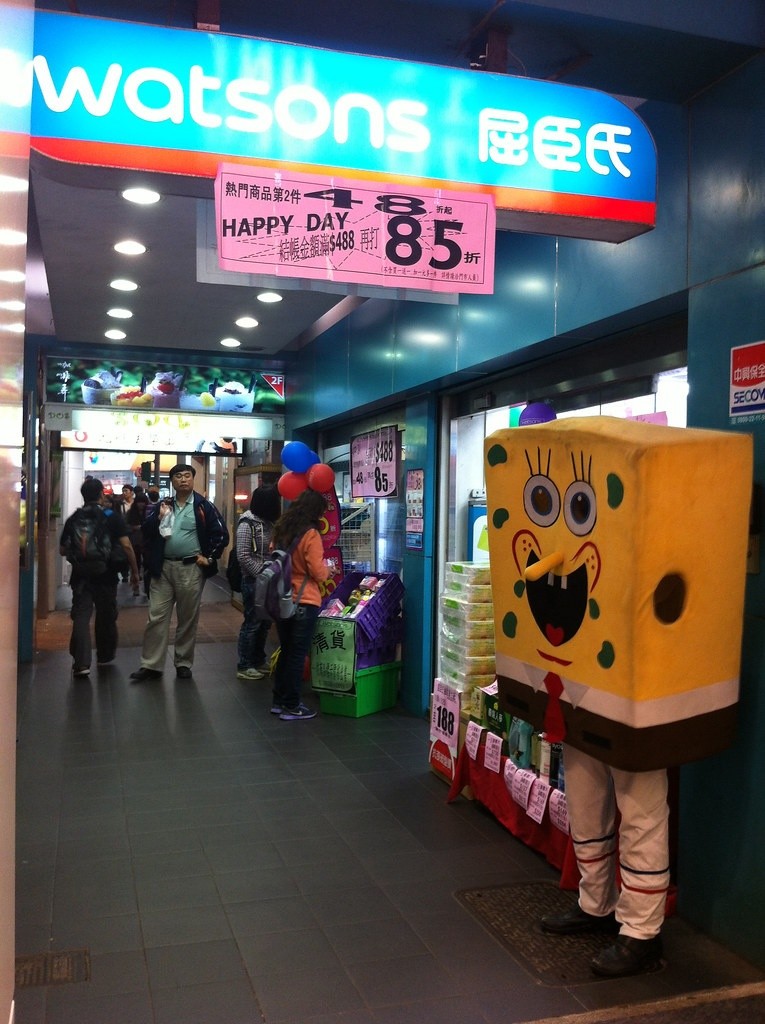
left=129, top=464, right=230, bottom=681
left=235, top=485, right=281, bottom=680
left=269, top=487, right=338, bottom=720
left=59, top=478, right=140, bottom=676
left=483, top=416, right=753, bottom=978
left=100, top=481, right=159, bottom=608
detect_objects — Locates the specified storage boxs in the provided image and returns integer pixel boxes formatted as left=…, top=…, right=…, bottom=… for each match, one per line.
left=312, top=568, right=408, bottom=719
left=429, top=691, right=511, bottom=802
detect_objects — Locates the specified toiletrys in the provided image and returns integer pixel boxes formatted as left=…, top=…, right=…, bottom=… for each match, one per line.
left=508, top=714, right=565, bottom=792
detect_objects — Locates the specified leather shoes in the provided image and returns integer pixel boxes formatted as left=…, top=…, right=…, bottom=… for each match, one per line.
left=590, top=926, right=665, bottom=976
left=539, top=902, right=618, bottom=937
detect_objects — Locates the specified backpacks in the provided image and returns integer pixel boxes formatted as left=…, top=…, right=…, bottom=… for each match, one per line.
left=66, top=507, right=117, bottom=566
left=254, top=533, right=309, bottom=621
left=225, top=517, right=255, bottom=593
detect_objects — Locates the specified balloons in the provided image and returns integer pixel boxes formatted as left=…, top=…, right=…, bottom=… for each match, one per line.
left=508, top=402, right=558, bottom=427
left=278, top=441, right=335, bottom=501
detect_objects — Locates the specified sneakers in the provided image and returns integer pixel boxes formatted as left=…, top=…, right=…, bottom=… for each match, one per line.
left=236, top=668, right=264, bottom=680
left=257, top=662, right=272, bottom=673
left=280, top=701, right=318, bottom=720
left=270, top=705, right=285, bottom=713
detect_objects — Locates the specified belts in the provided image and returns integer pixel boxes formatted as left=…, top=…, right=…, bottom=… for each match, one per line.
left=163, top=556, right=182, bottom=561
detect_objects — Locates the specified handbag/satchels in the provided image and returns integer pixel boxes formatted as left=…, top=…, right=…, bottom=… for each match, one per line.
left=158, top=508, right=174, bottom=539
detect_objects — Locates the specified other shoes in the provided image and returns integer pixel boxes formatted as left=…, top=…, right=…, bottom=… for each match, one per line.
left=96, top=658, right=113, bottom=665
left=129, top=668, right=163, bottom=681
left=177, top=666, right=192, bottom=679
left=72, top=669, right=90, bottom=676
left=133, top=589, right=138, bottom=596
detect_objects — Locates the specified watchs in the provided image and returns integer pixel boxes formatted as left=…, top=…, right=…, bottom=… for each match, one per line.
left=206, top=556, right=214, bottom=564
